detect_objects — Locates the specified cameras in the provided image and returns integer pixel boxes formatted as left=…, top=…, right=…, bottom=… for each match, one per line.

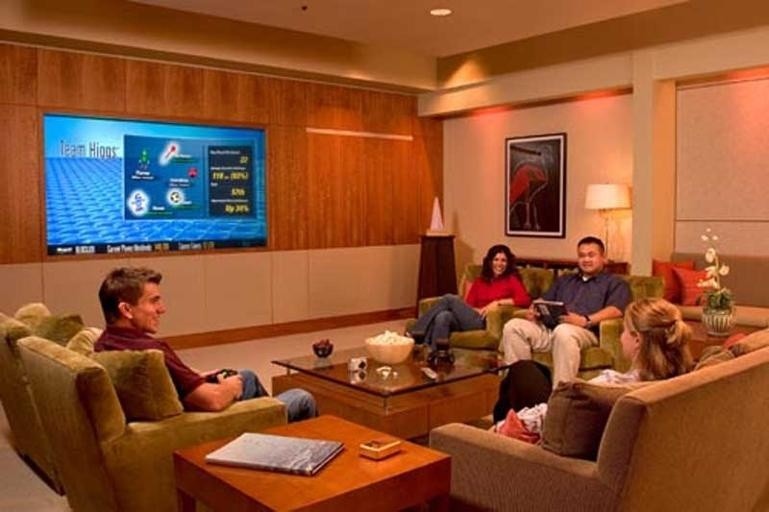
left=349, top=371, right=367, bottom=385
left=348, top=357, right=367, bottom=371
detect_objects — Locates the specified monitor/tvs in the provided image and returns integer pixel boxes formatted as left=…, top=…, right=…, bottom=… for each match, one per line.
left=42, top=112, right=268, bottom=257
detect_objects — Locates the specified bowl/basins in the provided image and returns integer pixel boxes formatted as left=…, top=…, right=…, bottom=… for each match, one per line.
left=365, top=335, right=415, bottom=366
left=312, top=343, right=333, bottom=358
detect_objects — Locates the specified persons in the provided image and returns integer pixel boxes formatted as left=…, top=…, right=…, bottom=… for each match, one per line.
left=497, top=236, right=632, bottom=404
left=94, top=265, right=318, bottom=424
left=488, top=294, right=691, bottom=438
left=403, top=244, right=533, bottom=352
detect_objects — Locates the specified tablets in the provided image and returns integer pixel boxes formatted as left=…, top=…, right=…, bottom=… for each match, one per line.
left=534, top=300, right=567, bottom=330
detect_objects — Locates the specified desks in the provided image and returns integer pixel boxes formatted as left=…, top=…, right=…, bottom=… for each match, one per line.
left=172, top=414, right=452, bottom=512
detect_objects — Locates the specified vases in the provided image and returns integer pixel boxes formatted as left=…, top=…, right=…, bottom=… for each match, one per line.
left=702, top=307, right=734, bottom=337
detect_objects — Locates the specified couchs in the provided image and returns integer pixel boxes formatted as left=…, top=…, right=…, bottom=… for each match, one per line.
left=502, top=274, right=665, bottom=381
left=0, top=302, right=85, bottom=512
left=671, top=251, right=769, bottom=327
left=16, top=326, right=288, bottom=512
left=402, top=264, right=553, bottom=372
left=430, top=327, right=768, bottom=512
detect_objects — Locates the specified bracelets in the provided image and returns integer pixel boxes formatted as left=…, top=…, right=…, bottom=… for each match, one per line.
left=582, top=314, right=592, bottom=330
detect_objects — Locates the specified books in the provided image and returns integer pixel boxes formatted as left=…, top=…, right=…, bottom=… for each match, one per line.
left=202, top=431, right=343, bottom=478
left=530, top=296, right=569, bottom=330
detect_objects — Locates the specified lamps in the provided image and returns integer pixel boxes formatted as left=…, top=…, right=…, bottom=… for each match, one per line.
left=584, top=182, right=633, bottom=258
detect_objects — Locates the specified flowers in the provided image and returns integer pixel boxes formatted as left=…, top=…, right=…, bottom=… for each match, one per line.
left=697, top=228, right=734, bottom=309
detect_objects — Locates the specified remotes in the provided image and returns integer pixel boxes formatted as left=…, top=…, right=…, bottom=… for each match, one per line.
left=420, top=367, right=439, bottom=380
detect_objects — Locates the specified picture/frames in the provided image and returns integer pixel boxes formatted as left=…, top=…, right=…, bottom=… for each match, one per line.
left=504, top=132, right=567, bottom=239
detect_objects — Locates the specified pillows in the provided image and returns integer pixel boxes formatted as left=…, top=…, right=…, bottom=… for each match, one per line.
left=673, top=267, right=707, bottom=307
left=652, top=259, right=697, bottom=305
left=66, top=328, right=185, bottom=421
